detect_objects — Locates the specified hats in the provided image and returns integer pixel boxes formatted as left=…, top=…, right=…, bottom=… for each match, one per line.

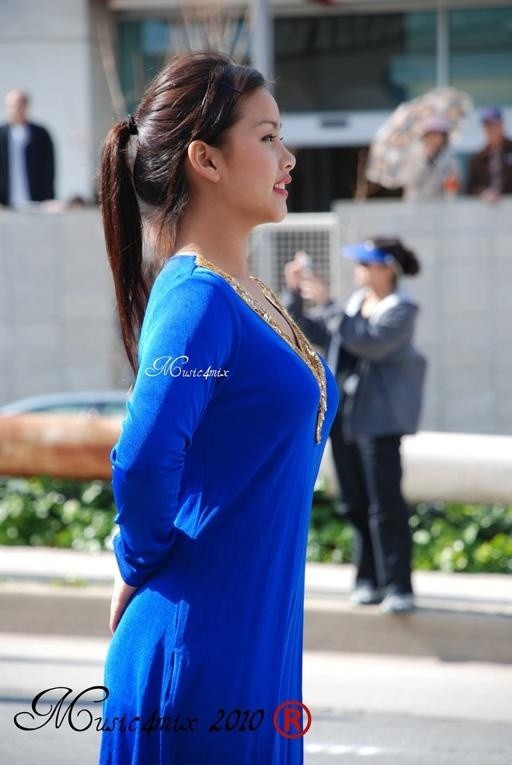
left=340, top=237, right=418, bottom=277
left=418, top=117, right=448, bottom=138
left=480, top=107, right=501, bottom=122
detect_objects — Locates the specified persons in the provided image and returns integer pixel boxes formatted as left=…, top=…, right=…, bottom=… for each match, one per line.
left=401, top=122, right=469, bottom=199
left=94, top=42, right=346, bottom=764
left=278, top=233, right=429, bottom=616
left=0, top=86, right=54, bottom=209
left=464, top=103, right=512, bottom=204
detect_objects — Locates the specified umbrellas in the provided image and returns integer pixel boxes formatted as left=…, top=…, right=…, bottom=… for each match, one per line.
left=363, top=87, right=474, bottom=192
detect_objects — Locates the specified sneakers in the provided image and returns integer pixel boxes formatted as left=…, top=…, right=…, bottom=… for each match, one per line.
left=351, top=585, right=415, bottom=614
